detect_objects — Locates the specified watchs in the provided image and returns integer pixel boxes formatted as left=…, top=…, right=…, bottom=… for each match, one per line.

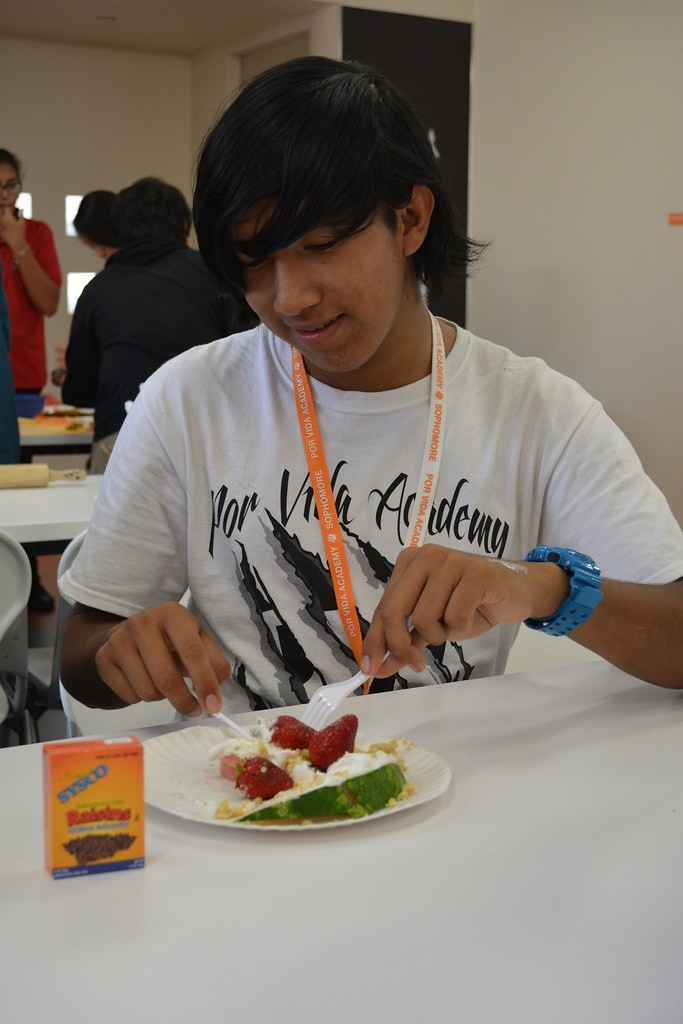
left=522, top=545, right=604, bottom=638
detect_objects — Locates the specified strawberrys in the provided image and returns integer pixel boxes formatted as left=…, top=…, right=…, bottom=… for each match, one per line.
left=304, top=713, right=358, bottom=773
left=270, top=715, right=319, bottom=750
left=235, top=757, right=294, bottom=800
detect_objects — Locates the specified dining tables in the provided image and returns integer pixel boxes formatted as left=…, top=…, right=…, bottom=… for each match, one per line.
left=18, top=417, right=95, bottom=456
left=0, top=656, right=683, bottom=1024
left=0, top=475, right=103, bottom=543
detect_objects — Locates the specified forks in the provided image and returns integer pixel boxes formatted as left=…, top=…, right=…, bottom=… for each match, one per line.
left=302, top=649, right=391, bottom=732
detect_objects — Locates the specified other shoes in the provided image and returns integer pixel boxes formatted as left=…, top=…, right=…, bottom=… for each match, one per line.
left=27, top=581, right=54, bottom=613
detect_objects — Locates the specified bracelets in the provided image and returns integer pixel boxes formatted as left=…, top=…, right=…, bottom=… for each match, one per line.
left=13, top=246, right=30, bottom=257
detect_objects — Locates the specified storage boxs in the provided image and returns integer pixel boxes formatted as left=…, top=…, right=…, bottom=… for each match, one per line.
left=36, top=730, right=147, bottom=883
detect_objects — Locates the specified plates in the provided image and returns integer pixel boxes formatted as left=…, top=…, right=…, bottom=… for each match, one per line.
left=143, top=726, right=452, bottom=832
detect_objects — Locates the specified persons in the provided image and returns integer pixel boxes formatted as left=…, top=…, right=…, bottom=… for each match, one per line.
left=56, top=53, right=683, bottom=723
left=61, top=176, right=261, bottom=475
left=0, top=145, right=63, bottom=464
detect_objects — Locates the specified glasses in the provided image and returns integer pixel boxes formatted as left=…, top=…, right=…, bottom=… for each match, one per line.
left=0, top=180, right=22, bottom=195
